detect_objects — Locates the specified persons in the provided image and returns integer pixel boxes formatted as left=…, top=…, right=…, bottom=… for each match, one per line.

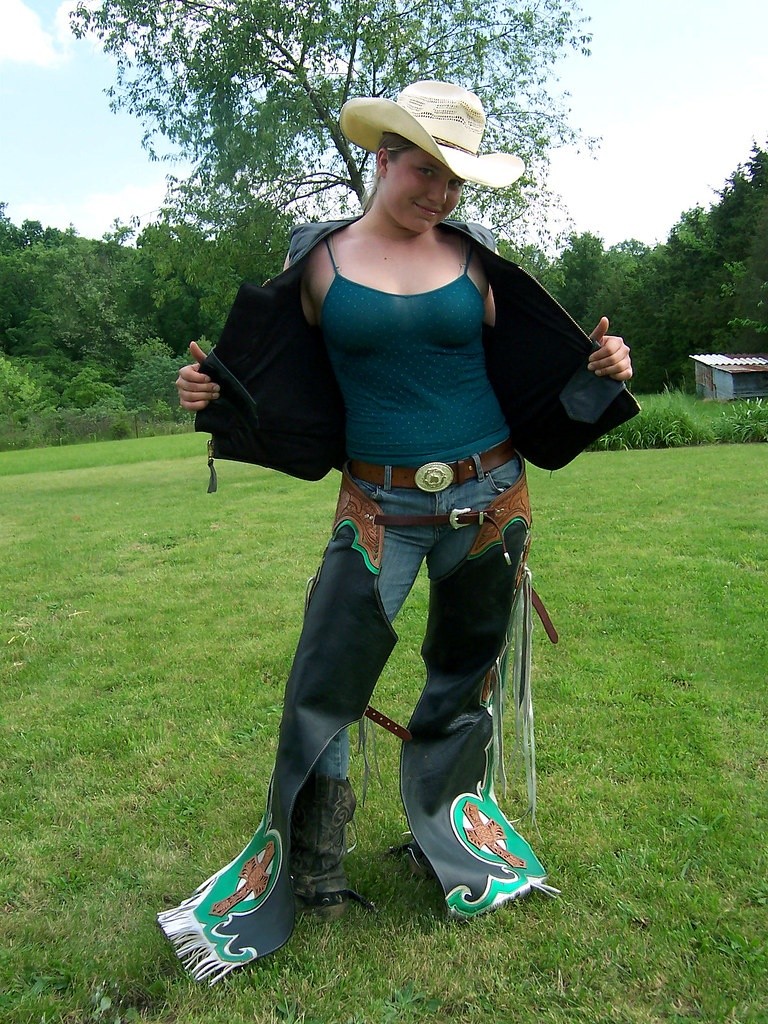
left=158, top=80, right=642, bottom=981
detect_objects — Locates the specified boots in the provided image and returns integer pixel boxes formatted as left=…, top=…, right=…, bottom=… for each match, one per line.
left=289, top=773, right=378, bottom=919
left=401, top=840, right=436, bottom=881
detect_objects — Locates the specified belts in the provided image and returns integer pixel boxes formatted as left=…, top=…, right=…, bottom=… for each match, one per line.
left=348, top=437, right=515, bottom=492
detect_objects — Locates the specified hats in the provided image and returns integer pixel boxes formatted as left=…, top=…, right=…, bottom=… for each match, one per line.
left=339, top=80, right=525, bottom=188
